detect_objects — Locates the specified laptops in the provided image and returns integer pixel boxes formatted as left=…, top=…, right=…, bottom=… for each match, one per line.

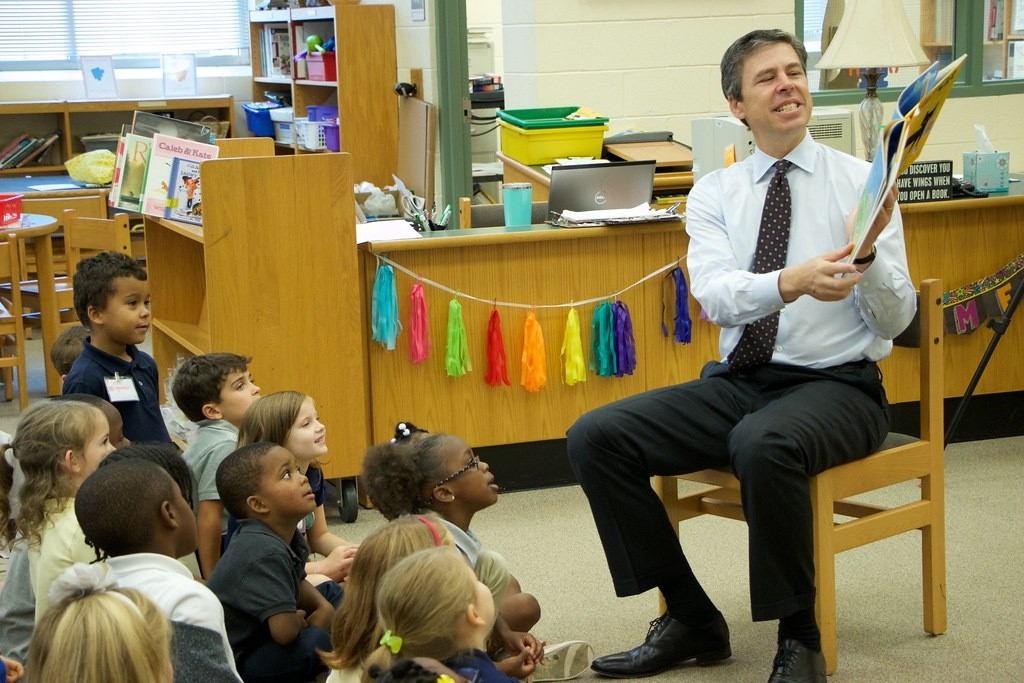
left=546, top=159, right=657, bottom=223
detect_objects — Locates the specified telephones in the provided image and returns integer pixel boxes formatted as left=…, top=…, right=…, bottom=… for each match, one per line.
left=951, top=177, right=974, bottom=196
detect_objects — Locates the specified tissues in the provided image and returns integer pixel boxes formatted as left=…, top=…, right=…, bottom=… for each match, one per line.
left=962, top=123, right=1011, bottom=193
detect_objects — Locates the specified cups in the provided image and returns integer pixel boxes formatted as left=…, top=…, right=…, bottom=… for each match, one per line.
left=502, top=182, right=533, bottom=226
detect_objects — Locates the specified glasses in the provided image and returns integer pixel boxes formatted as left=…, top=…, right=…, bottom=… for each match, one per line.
left=425, top=452, right=480, bottom=508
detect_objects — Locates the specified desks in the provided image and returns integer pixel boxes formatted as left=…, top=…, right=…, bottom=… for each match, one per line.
left=0, top=212, right=64, bottom=396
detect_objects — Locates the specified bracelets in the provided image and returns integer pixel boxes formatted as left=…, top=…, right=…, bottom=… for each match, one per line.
left=853, top=244, right=878, bottom=268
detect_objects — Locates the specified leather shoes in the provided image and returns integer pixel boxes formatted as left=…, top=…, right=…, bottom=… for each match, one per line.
left=767, top=639, right=828, bottom=683
left=591, top=610, right=732, bottom=678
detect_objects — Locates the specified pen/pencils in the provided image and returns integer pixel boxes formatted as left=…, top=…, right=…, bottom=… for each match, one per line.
left=415, top=213, right=431, bottom=231
left=438, top=203, right=452, bottom=226
left=430, top=202, right=436, bottom=222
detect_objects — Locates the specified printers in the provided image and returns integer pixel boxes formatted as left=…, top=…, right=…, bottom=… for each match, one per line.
left=690, top=106, right=856, bottom=186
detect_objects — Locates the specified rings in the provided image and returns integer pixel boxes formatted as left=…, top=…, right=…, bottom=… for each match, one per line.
left=886, top=207, right=895, bottom=210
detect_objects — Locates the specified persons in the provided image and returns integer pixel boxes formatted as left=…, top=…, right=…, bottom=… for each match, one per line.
left=0, top=252, right=592, bottom=683
left=357, top=542, right=524, bottom=683
left=566, top=28, right=918, bottom=683
left=25, top=560, right=181, bottom=683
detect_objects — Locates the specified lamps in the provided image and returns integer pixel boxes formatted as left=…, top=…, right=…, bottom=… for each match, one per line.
left=814, top=2, right=931, bottom=162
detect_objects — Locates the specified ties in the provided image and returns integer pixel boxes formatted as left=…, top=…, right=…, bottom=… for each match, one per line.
left=725, top=161, right=792, bottom=381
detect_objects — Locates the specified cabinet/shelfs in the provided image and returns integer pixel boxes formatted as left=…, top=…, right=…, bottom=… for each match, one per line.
left=0, top=96, right=237, bottom=175
left=143, top=137, right=367, bottom=523
left=249, top=5, right=399, bottom=209
left=919, top=0, right=1024, bottom=80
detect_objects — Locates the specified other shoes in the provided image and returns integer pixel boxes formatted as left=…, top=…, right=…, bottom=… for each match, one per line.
left=494, top=638, right=595, bottom=682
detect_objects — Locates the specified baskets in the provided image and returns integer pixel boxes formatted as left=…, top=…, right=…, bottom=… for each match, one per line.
left=187, top=111, right=230, bottom=139
left=0, top=193, right=24, bottom=226
left=294, top=119, right=333, bottom=150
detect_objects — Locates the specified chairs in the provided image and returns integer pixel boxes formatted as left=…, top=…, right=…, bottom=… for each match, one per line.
left=0, top=188, right=133, bottom=411
left=654, top=278, right=946, bottom=676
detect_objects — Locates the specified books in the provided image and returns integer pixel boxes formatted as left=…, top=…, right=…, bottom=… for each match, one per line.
left=258, top=24, right=288, bottom=78
left=0, top=129, right=59, bottom=169
left=468, top=70, right=505, bottom=102
left=106, top=108, right=219, bottom=226
left=839, top=51, right=969, bottom=285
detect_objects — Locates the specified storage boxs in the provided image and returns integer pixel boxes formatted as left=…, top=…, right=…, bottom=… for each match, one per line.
left=306, top=52, right=337, bottom=81
left=497, top=107, right=609, bottom=165
left=269, top=105, right=340, bottom=151
left=242, top=103, right=282, bottom=137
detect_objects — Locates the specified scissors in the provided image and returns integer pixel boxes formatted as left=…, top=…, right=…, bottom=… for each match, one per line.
left=401, top=195, right=424, bottom=218
